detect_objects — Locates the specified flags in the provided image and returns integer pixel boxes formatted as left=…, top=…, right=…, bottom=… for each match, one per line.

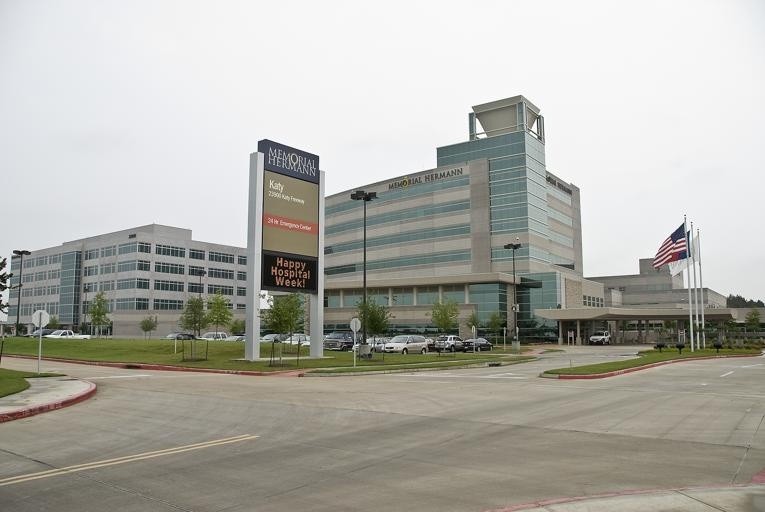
left=652, top=223, right=687, bottom=269
left=667, top=236, right=698, bottom=277
left=687, top=231, right=692, bottom=256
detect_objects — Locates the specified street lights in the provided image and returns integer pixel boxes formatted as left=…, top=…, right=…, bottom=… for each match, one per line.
left=198, top=270, right=206, bottom=336
left=504, top=242, right=521, bottom=340
left=351, top=191, right=377, bottom=344
left=13, top=249, right=31, bottom=335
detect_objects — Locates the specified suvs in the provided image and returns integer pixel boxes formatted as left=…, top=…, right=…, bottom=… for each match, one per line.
left=589, top=330, right=612, bottom=345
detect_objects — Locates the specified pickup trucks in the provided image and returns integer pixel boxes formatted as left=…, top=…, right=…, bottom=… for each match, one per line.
left=42, top=330, right=91, bottom=340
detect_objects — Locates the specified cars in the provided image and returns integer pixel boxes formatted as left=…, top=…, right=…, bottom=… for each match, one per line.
left=23, top=329, right=52, bottom=337
